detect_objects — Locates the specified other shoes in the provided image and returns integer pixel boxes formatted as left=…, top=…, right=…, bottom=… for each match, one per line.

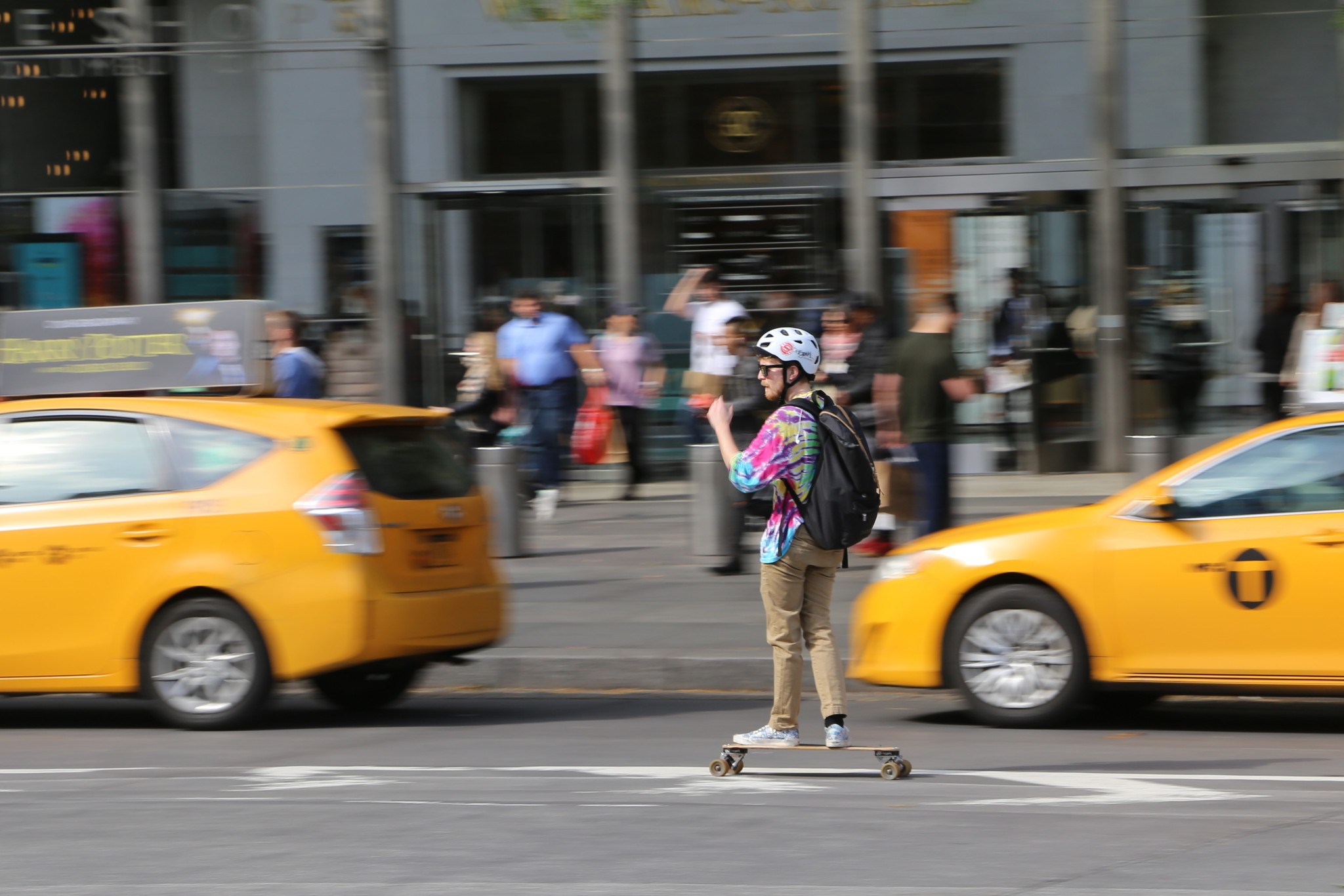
left=855, top=540, right=892, bottom=557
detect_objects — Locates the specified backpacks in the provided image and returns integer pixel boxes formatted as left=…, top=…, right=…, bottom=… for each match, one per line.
left=781, top=391, right=882, bottom=569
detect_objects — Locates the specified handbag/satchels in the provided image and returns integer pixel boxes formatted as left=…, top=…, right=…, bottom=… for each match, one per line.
left=570, top=387, right=616, bottom=464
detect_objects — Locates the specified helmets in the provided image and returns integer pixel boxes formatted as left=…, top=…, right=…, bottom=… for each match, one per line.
left=756, top=327, right=820, bottom=377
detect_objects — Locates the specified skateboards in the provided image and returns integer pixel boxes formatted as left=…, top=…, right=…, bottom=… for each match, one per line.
left=709, top=744, right=911, bottom=781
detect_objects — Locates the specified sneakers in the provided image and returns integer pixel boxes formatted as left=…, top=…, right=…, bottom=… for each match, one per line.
left=824, top=724, right=853, bottom=748
left=733, top=724, right=800, bottom=747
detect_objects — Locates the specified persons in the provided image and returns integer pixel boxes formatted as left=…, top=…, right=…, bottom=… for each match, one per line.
left=708, top=329, right=885, bottom=749
left=259, top=313, right=325, bottom=409
left=403, top=261, right=1342, bottom=511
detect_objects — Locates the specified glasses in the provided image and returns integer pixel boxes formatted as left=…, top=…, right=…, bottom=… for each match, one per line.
left=757, top=364, right=786, bottom=377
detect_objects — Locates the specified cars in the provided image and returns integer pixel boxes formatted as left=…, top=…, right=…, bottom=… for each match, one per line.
left=0, top=398, right=512, bottom=729
left=843, top=410, right=1344, bottom=729
